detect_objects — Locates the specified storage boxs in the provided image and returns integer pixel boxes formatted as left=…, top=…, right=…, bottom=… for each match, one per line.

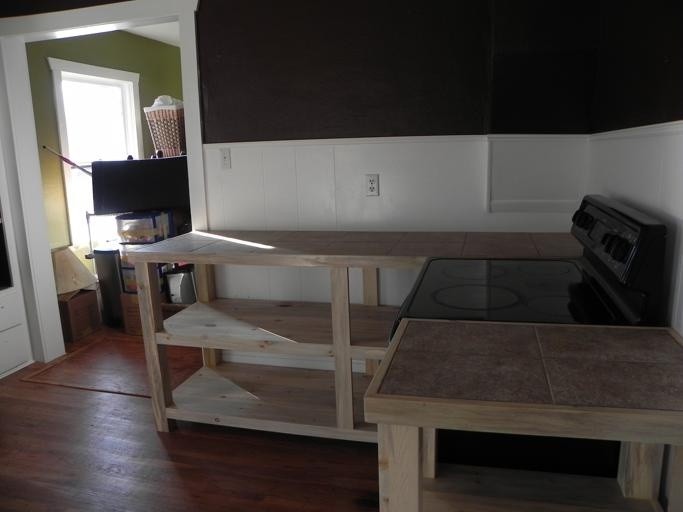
left=114, top=213, right=170, bottom=295
left=58, top=290, right=99, bottom=344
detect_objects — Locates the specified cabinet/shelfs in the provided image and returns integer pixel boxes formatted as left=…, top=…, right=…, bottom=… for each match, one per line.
left=123, top=228, right=583, bottom=445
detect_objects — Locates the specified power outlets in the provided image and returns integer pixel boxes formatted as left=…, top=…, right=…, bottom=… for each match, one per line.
left=364, top=174, right=379, bottom=196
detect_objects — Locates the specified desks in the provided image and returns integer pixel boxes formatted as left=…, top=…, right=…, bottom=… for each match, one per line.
left=363, top=317, right=682, bottom=511
